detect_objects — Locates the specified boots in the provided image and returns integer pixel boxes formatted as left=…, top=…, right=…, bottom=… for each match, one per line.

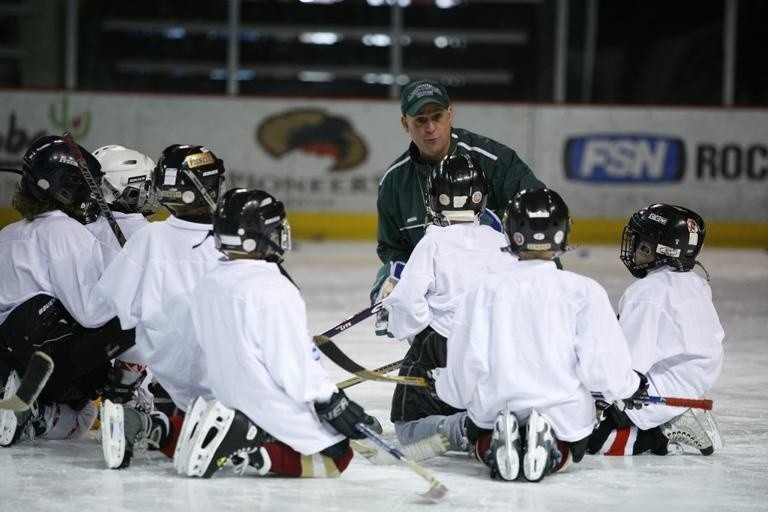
left=660, top=408, right=714, bottom=456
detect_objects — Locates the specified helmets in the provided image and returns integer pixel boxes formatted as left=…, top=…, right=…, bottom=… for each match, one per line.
left=620, top=202, right=707, bottom=278
left=501, top=188, right=570, bottom=253
left=13, top=135, right=292, bottom=262
left=424, top=153, right=487, bottom=224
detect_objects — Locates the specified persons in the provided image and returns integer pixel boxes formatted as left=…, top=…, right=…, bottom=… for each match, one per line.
left=0, top=135, right=104, bottom=443
left=192, top=186, right=373, bottom=478
left=385, top=151, right=513, bottom=449
left=467, top=189, right=649, bottom=480
left=585, top=202, right=728, bottom=457
left=369, top=79, right=564, bottom=453
left=115, top=142, right=230, bottom=467
left=83, top=144, right=156, bottom=266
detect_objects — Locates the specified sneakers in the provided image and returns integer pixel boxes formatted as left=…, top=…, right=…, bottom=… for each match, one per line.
left=114, top=406, right=141, bottom=473
left=200, top=408, right=248, bottom=479
left=492, top=409, right=556, bottom=480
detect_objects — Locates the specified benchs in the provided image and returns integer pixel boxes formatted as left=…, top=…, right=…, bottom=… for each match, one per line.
left=80, top=10, right=529, bottom=87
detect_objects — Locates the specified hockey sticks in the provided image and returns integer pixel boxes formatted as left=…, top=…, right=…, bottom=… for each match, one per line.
left=312, top=334, right=713, bottom=412
left=352, top=422, right=449, bottom=503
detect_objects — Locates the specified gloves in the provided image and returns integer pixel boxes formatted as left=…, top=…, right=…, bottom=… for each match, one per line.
left=314, top=389, right=382, bottom=440
left=622, top=372, right=650, bottom=410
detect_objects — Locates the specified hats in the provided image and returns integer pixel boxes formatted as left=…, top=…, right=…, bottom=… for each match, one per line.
left=400, top=79, right=449, bottom=117
